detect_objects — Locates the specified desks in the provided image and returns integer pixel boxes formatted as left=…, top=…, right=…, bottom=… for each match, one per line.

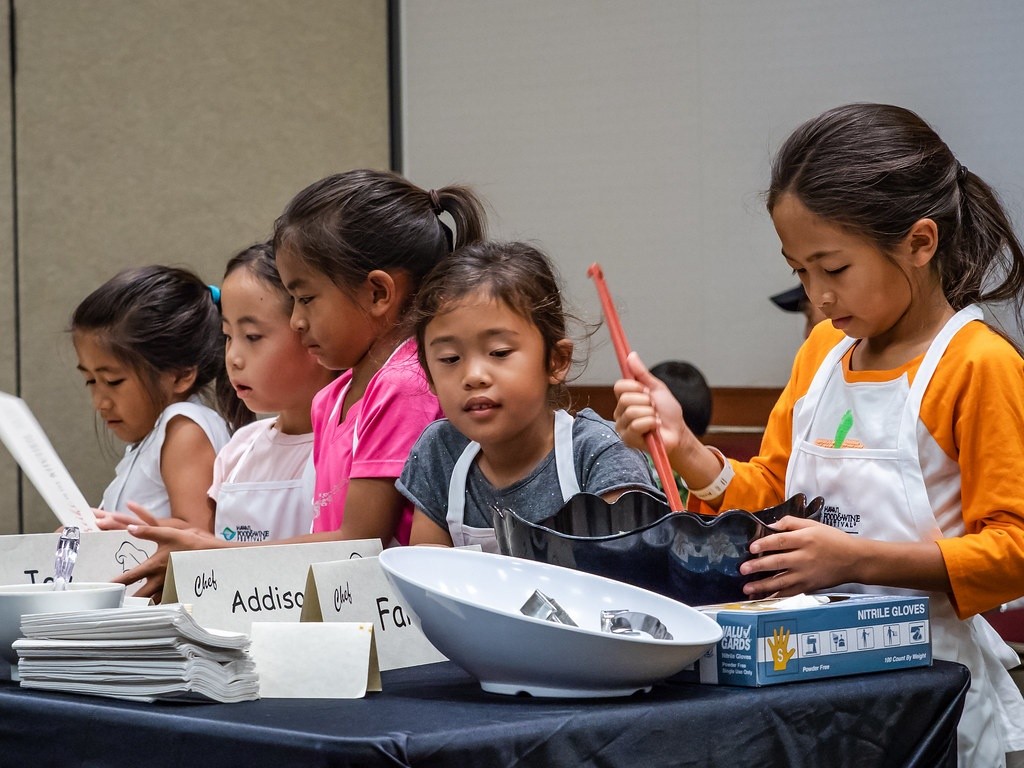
left=0, top=650, right=970, bottom=768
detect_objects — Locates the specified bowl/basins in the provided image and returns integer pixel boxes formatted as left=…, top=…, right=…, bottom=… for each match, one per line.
left=489, top=489, right=825, bottom=607
left=0, top=582, right=126, bottom=665
left=378, top=546, right=723, bottom=700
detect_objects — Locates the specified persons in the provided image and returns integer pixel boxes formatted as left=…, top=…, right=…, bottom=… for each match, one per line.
left=51, top=102, right=1023, bottom=768
left=648, top=360, right=713, bottom=441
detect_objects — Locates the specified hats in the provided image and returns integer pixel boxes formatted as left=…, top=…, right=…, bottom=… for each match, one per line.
left=770, top=282, right=810, bottom=313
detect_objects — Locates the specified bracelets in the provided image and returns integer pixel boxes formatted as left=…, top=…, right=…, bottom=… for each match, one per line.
left=681, top=446, right=734, bottom=502
